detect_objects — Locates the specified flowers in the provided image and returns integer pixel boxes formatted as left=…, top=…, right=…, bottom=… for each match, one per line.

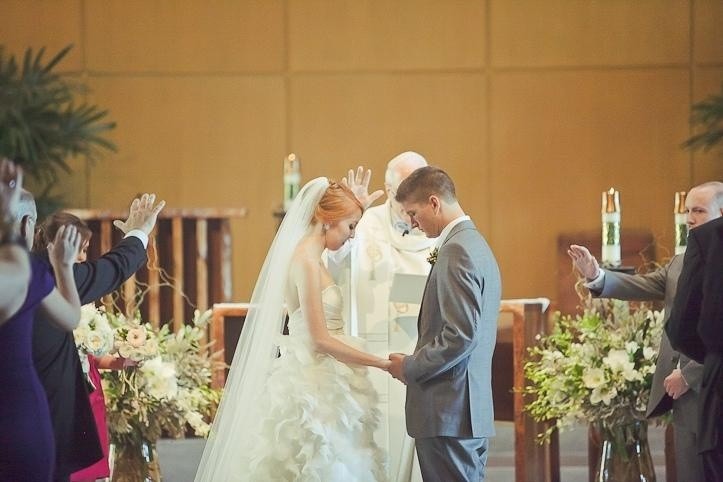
left=506, top=284, right=678, bottom=482
left=72, top=299, right=231, bottom=481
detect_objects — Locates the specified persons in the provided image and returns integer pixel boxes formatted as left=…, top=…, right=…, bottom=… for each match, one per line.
left=0, top=157, right=23, bottom=189
left=0, top=184, right=83, bottom=482
left=0, top=187, right=167, bottom=482
left=30, top=211, right=142, bottom=481
left=663, top=214, right=722, bottom=481
left=249, top=175, right=394, bottom=482
left=565, top=179, right=722, bottom=481
left=316, top=150, right=441, bottom=482
left=387, top=164, right=501, bottom=481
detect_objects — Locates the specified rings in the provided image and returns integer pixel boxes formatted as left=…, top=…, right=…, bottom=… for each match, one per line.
left=8, top=179, right=15, bottom=189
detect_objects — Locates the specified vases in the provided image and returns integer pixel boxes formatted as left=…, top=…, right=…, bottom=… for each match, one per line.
left=597, top=420, right=655, bottom=482
